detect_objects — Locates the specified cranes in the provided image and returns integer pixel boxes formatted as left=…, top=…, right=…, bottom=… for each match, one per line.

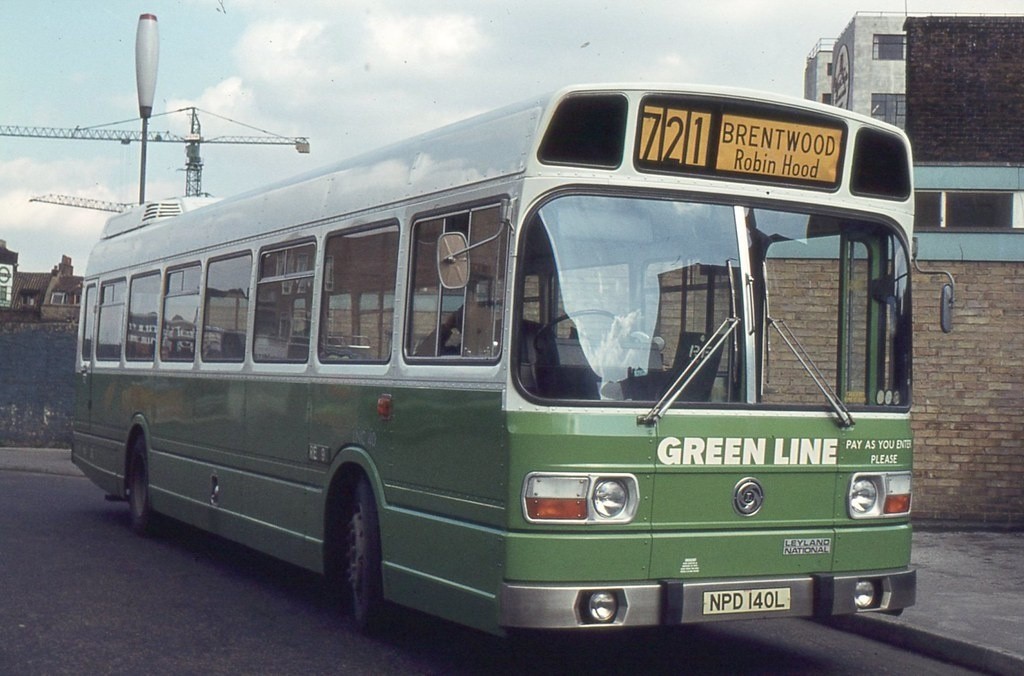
left=0, top=106, right=313, bottom=194
left=28, top=194, right=132, bottom=214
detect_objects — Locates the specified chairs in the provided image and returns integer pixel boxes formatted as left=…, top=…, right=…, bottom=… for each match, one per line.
left=124, top=312, right=567, bottom=386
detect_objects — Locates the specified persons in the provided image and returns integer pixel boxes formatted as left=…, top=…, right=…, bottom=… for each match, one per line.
left=413, top=300, right=493, bottom=358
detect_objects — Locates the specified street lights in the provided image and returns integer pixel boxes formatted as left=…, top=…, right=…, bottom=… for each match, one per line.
left=132, top=11, right=160, bottom=205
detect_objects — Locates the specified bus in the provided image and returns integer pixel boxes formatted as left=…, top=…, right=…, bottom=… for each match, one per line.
left=63, top=82, right=959, bottom=645
